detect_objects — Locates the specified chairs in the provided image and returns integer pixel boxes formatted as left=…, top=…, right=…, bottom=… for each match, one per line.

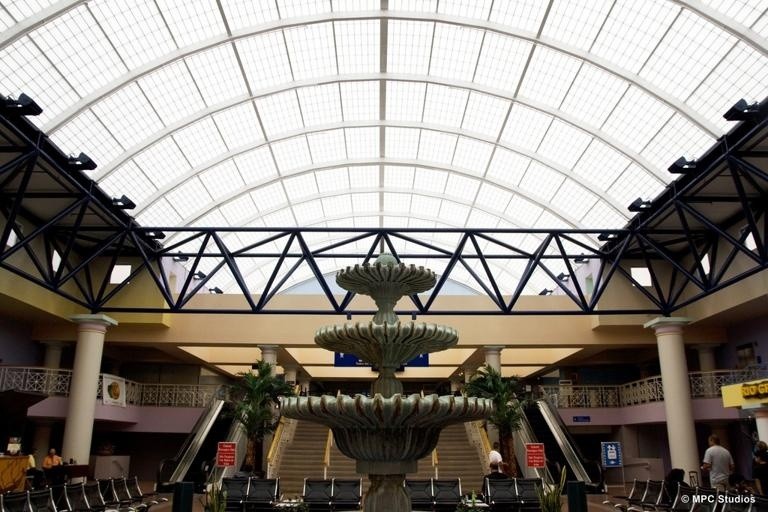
left=0, top=491, right=32, bottom=512
left=603, top=478, right=649, bottom=512
left=664, top=482, right=699, bottom=512
left=301, top=478, right=334, bottom=512
left=485, top=478, right=524, bottom=512
left=124, top=476, right=158, bottom=512
left=220, top=477, right=250, bottom=512
left=28, top=487, right=57, bottom=512
left=721, top=492, right=754, bottom=512
left=112, top=477, right=148, bottom=512
left=50, top=486, right=72, bottom=512
left=64, top=484, right=90, bottom=512
left=690, top=487, right=719, bottom=512
left=329, top=479, right=362, bottom=512
left=98, top=478, right=133, bottom=512
left=432, top=478, right=464, bottom=512
left=83, top=482, right=106, bottom=512
left=403, top=478, right=436, bottom=512
left=240, top=477, right=280, bottom=512
left=514, top=478, right=547, bottom=512
left=615, top=480, right=664, bottom=512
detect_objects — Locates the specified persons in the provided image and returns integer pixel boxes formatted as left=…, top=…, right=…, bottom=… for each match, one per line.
left=26, top=447, right=47, bottom=491
left=43, top=449, right=66, bottom=495
left=752, top=450, right=768, bottom=497
left=752, top=442, right=768, bottom=468
left=700, top=434, right=734, bottom=512
left=489, top=442, right=509, bottom=474
